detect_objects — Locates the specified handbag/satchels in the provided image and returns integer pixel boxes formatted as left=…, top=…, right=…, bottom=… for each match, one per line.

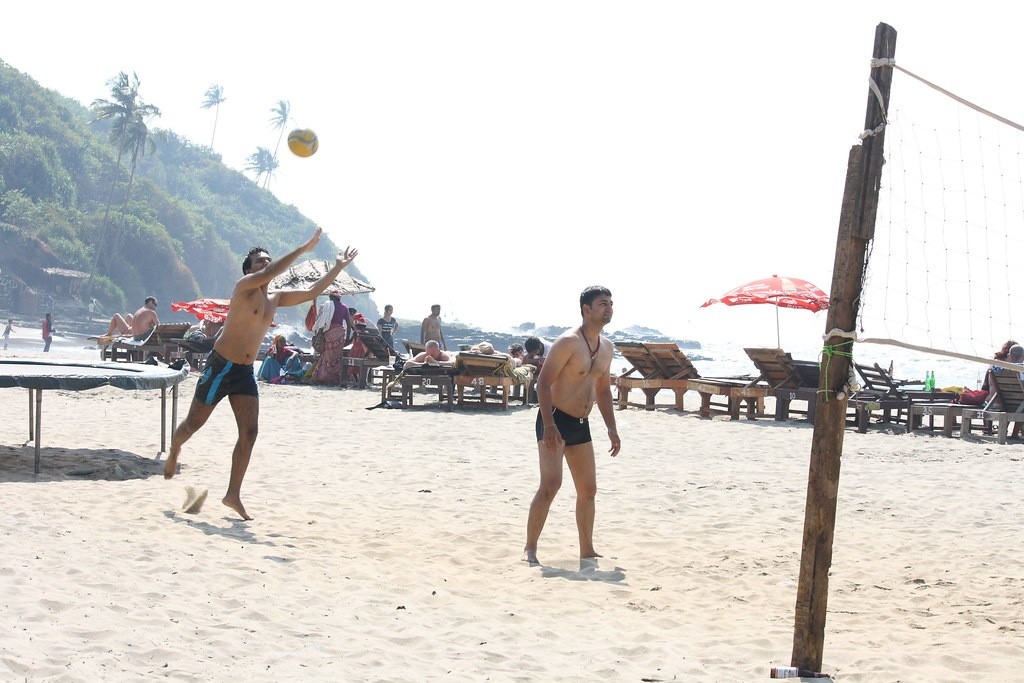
left=168, top=359, right=190, bottom=370
left=959, top=387, right=989, bottom=405
left=312, top=327, right=325, bottom=352
left=305, top=304, right=317, bottom=331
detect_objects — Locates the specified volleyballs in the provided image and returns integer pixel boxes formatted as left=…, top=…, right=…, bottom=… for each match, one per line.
left=287, top=127, right=319, bottom=158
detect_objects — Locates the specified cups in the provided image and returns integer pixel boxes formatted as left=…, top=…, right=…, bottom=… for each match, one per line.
left=389, top=356, right=396, bottom=366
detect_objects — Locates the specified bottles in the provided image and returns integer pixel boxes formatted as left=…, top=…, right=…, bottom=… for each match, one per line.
left=930, top=371, right=935, bottom=392
left=976, top=371, right=982, bottom=390
left=771, top=667, right=830, bottom=678
left=925, top=370, right=930, bottom=392
left=889, top=360, right=894, bottom=378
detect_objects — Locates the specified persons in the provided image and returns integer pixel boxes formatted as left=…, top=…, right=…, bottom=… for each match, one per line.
left=256, top=292, right=553, bottom=405
left=2, top=319, right=14, bottom=351
left=521, top=284, right=620, bottom=564
left=981, top=341, right=1024, bottom=441
left=164, top=227, right=358, bottom=520
left=131, top=296, right=160, bottom=346
left=42, top=312, right=55, bottom=352
left=101, top=312, right=134, bottom=337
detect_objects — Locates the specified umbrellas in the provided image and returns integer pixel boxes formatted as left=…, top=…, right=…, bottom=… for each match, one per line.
left=170, top=298, right=276, bottom=327
left=267, top=260, right=375, bottom=324
left=700, top=274, right=831, bottom=350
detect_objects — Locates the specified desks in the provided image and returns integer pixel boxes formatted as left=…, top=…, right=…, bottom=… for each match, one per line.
left=905, top=389, right=958, bottom=432
left=0, top=356, right=189, bottom=474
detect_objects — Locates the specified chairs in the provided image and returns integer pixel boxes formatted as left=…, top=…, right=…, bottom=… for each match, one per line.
left=88, top=321, right=1024, bottom=444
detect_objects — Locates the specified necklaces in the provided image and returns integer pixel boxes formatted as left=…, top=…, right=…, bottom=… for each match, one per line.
left=579, top=325, right=600, bottom=359
